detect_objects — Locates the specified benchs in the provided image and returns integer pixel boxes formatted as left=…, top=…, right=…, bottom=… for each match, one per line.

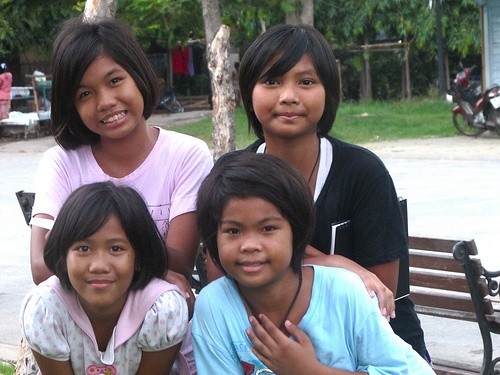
left=408, top=235, right=500, bottom=375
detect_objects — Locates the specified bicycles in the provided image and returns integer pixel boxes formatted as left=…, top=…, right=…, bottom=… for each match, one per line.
left=159, top=84, right=184, bottom=114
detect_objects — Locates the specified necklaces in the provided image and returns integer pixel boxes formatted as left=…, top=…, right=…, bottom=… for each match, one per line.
left=308, top=154, right=319, bottom=182
left=245, top=270, right=302, bottom=332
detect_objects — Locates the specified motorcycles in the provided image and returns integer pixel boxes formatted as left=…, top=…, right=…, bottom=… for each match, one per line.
left=444, top=60, right=500, bottom=139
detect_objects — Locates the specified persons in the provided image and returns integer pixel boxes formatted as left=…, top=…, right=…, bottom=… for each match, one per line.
left=204, top=22, right=428, bottom=362
left=0, top=61, right=12, bottom=122
left=29, top=18, right=216, bottom=375
left=22, top=182, right=189, bottom=375
left=191, top=150, right=441, bottom=374
